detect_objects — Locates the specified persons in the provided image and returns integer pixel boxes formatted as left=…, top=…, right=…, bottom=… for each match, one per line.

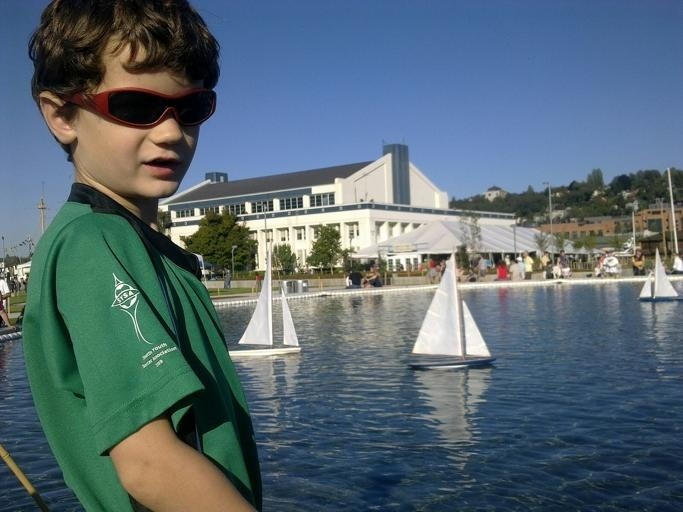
left=0, top=266, right=29, bottom=331
left=345, top=259, right=382, bottom=289
left=631, top=248, right=683, bottom=275
left=456, top=250, right=586, bottom=282
left=589, top=251, right=623, bottom=278
left=254, top=272, right=261, bottom=288
left=396, top=257, right=446, bottom=285
left=294, top=262, right=315, bottom=275
left=223, top=266, right=232, bottom=288
left=21, top=1, right=264, bottom=512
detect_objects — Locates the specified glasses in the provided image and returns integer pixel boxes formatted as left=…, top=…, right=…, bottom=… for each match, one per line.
left=61, top=88, right=216, bottom=127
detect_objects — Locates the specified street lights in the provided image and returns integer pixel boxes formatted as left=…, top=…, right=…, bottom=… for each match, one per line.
left=542, top=180, right=555, bottom=267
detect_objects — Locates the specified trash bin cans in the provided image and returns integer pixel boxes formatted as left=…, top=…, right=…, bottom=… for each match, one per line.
left=283, top=279, right=308, bottom=294
